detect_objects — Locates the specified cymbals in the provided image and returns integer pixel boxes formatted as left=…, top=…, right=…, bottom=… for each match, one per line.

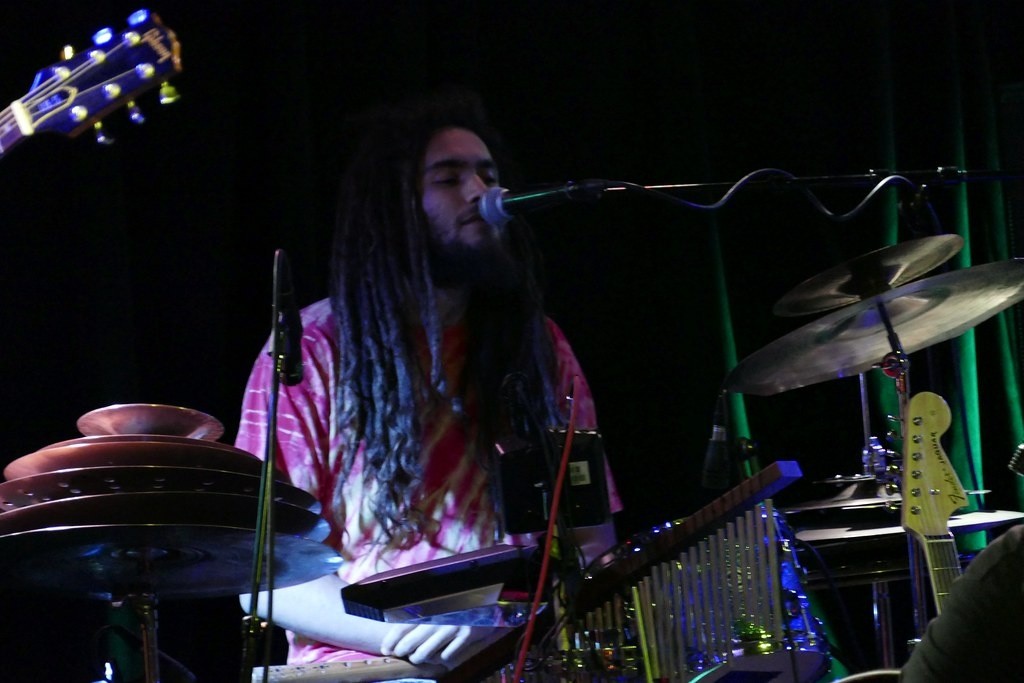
left=728, top=255, right=1024, bottom=395
left=778, top=483, right=1024, bottom=588
left=0, top=401, right=346, bottom=605
left=772, top=227, right=966, bottom=320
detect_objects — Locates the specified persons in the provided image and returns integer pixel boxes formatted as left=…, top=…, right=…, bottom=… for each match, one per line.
left=233, top=98, right=621, bottom=665
left=898, top=522, right=1024, bottom=683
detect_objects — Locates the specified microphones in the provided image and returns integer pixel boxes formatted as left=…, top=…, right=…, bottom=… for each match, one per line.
left=702, top=394, right=728, bottom=489
left=478, top=180, right=582, bottom=227
left=274, top=248, right=305, bottom=386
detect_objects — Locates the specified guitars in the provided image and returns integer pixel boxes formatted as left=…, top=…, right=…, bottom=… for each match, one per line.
left=0, top=8, right=189, bottom=155
left=895, top=388, right=968, bottom=614
